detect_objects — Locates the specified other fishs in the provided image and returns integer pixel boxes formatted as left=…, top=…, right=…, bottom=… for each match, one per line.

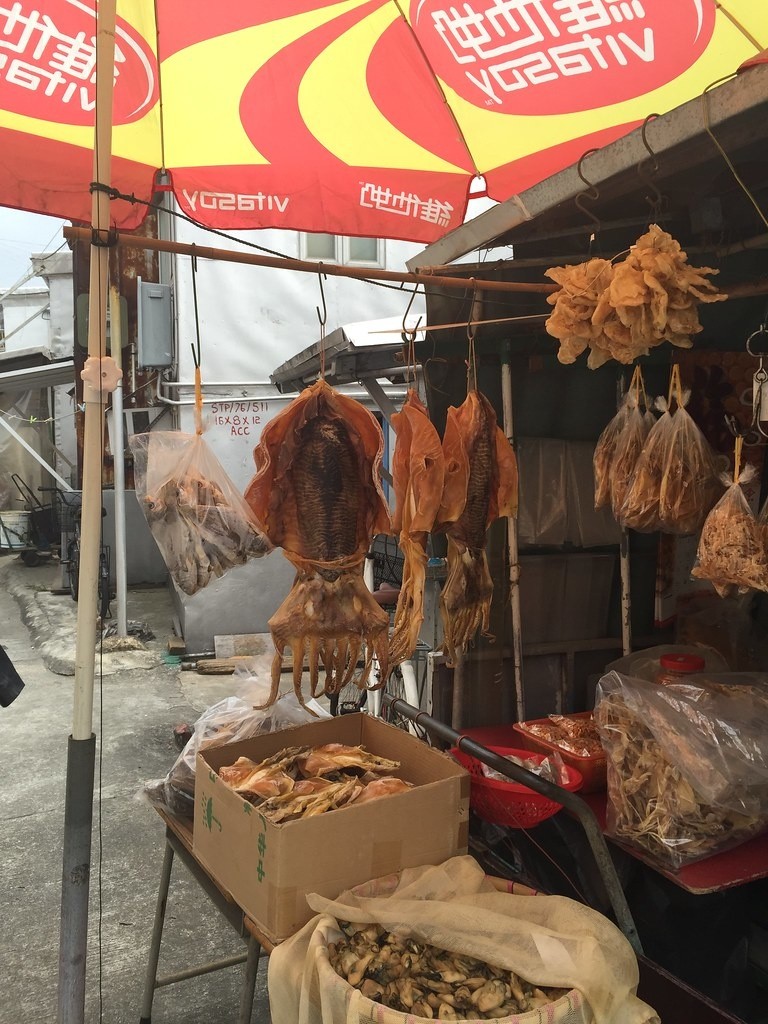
left=142, top=473, right=269, bottom=594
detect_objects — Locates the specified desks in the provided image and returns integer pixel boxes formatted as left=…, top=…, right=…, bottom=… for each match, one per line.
left=138, top=782, right=277, bottom=1024
left=462, top=710, right=768, bottom=930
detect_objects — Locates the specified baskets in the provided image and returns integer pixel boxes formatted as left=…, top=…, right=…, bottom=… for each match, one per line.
left=514, top=710, right=611, bottom=796
left=444, top=746, right=582, bottom=827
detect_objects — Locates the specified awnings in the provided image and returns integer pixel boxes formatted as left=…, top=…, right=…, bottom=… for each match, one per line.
left=0, top=357, right=78, bottom=492
left=268, top=312, right=426, bottom=434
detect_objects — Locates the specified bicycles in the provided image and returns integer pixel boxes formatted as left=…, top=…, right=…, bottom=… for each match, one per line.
left=37, top=484, right=115, bottom=618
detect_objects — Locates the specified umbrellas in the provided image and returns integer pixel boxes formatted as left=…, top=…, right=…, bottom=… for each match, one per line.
left=0, top=0, right=766, bottom=1024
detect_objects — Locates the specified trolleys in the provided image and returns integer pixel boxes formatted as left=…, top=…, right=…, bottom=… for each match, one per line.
left=0, top=474, right=60, bottom=567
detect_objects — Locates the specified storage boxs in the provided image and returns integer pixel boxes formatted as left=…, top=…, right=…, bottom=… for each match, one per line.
left=194, top=711, right=472, bottom=942
